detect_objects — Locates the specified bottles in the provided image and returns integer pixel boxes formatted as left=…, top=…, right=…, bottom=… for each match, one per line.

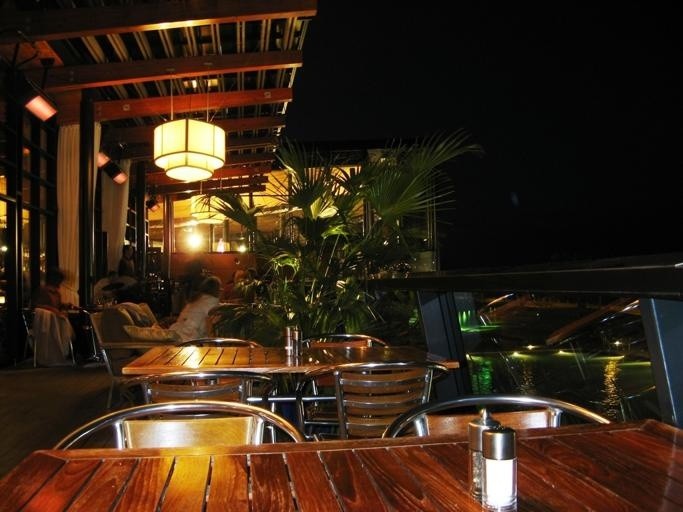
left=482, top=430, right=518, bottom=507
left=469, top=408, right=500, bottom=499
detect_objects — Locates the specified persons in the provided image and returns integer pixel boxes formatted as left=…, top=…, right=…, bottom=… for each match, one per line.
left=26, top=242, right=253, bottom=367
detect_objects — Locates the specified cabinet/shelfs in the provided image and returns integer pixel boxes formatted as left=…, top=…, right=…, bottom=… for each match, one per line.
left=124, top=207, right=149, bottom=252
